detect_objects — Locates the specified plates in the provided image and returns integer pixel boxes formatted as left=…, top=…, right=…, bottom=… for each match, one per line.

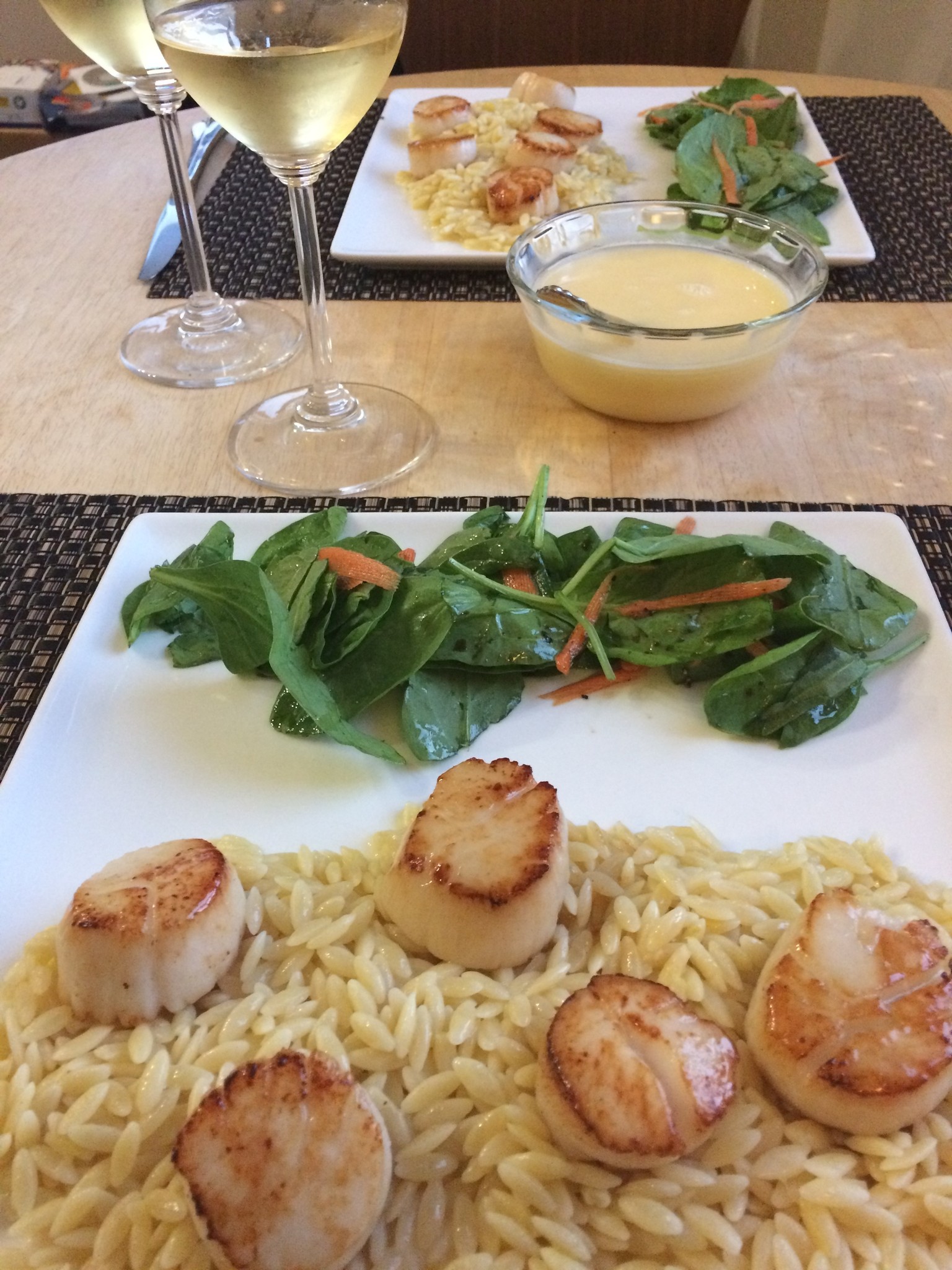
left=330, top=86, right=875, bottom=266
left=1, top=513, right=952, bottom=1269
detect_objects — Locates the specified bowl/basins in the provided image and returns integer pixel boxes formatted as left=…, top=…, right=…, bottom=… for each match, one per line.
left=506, top=199, right=830, bottom=425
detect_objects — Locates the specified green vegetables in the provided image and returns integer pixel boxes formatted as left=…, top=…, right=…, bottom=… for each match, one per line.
left=641, top=75, right=839, bottom=259
left=119, top=463, right=930, bottom=766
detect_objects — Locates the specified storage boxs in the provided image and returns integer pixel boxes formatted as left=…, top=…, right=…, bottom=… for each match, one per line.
left=37, top=78, right=143, bottom=136
left=0, top=59, right=57, bottom=124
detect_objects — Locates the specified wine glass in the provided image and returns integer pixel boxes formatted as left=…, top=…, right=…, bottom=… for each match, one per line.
left=38, top=1, right=309, bottom=388
left=142, top=1, right=439, bottom=497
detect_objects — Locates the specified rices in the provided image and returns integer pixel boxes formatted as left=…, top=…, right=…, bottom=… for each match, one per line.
left=0, top=798, right=952, bottom=1270
left=394, top=96, right=639, bottom=254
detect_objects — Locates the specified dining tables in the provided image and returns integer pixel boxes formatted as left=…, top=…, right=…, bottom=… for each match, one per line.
left=0, top=65, right=952, bottom=1269
